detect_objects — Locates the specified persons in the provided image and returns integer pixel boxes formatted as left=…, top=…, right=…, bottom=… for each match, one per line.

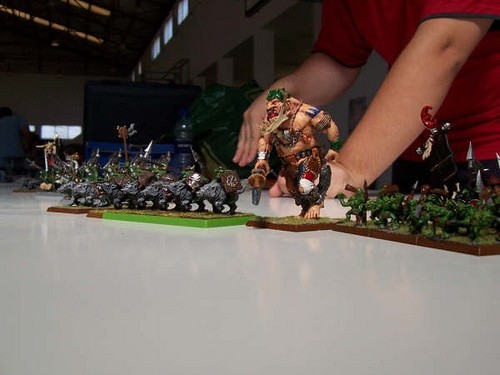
left=0, top=105, right=36, bottom=179
left=231, top=0, right=500, bottom=200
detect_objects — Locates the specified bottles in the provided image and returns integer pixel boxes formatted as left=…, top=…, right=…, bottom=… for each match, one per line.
left=173, top=111, right=195, bottom=159
